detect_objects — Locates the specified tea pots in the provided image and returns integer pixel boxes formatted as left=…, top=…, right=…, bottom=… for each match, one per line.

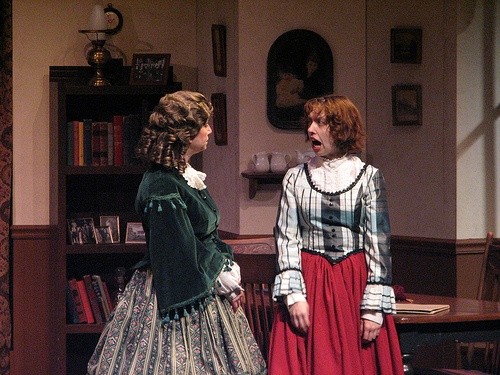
left=295, top=149, right=316, bottom=165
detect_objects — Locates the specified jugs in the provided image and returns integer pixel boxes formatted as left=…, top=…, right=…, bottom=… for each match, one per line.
left=270, top=150, right=291, bottom=172
left=251, top=152, right=270, bottom=172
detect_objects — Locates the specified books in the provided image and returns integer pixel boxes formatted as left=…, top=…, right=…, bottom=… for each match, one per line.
left=396, top=303, right=450, bottom=314
left=67, top=115, right=132, bottom=168
left=66, top=274, right=124, bottom=324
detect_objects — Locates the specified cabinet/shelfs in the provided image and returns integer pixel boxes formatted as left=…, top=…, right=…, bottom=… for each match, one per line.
left=49, top=65, right=183, bottom=375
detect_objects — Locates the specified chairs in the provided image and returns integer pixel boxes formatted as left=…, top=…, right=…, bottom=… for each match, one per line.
left=413, top=231, right=500, bottom=375
left=222, top=253, right=282, bottom=363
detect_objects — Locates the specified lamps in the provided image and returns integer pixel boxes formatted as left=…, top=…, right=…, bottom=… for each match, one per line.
left=78, top=4, right=114, bottom=87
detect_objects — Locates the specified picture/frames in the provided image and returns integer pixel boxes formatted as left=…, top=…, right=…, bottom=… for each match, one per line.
left=129, top=52, right=171, bottom=86
left=211, top=92, right=227, bottom=146
left=67, top=214, right=146, bottom=245
left=390, top=27, right=422, bottom=64
left=391, top=85, right=422, bottom=126
left=211, top=24, right=226, bottom=77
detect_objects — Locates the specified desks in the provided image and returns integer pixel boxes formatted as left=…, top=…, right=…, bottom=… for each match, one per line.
left=241, top=290, right=500, bottom=375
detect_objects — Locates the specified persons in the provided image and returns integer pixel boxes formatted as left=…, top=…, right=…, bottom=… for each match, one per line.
left=267, top=95, right=404, bottom=375
left=87, top=91, right=268, bottom=375
left=275, top=60, right=327, bottom=107
